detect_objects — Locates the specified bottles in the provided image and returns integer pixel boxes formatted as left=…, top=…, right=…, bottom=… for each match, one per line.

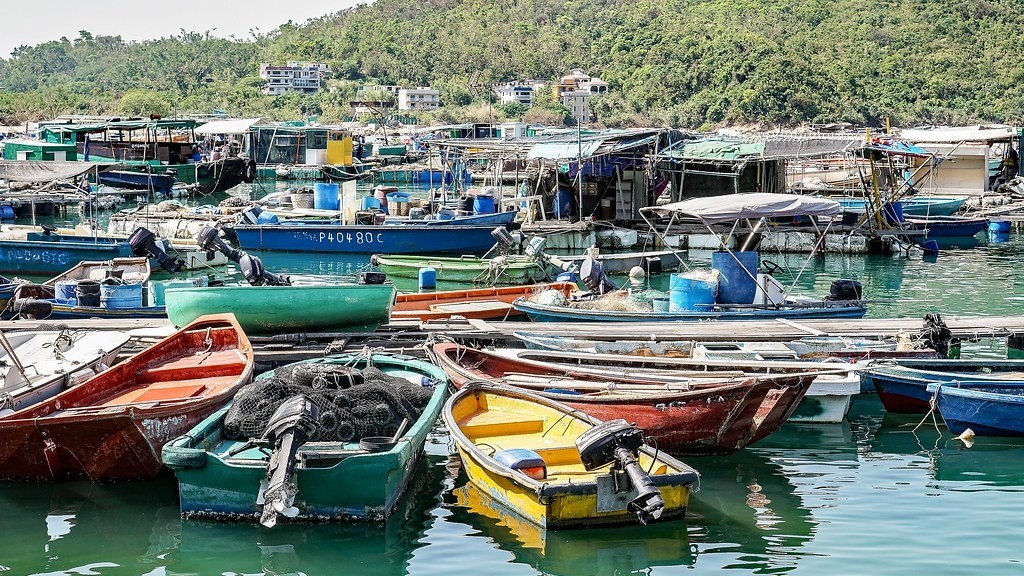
left=67, top=368, right=95, bottom=386
left=96, top=363, right=110, bottom=372
left=948, top=337, right=961, bottom=358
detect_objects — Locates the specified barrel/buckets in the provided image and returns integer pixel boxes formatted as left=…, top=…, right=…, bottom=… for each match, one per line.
left=905, top=172, right=911, bottom=185
left=0, top=286, right=16, bottom=320
left=882, top=201, right=902, bottom=225
left=186, top=152, right=200, bottom=163
left=553, top=187, right=574, bottom=220
left=554, top=272, right=577, bottom=283
left=100, top=285, right=142, bottom=309
left=419, top=266, right=436, bottom=289
left=55, top=284, right=77, bottom=305
left=735, top=233, right=762, bottom=251
left=922, top=240, right=938, bottom=252
left=712, top=251, right=757, bottom=309
left=988, top=221, right=1012, bottom=232
left=257, top=211, right=278, bottom=225
left=437, top=208, right=455, bottom=220
left=147, top=276, right=208, bottom=307
left=669, top=273, right=717, bottom=312
left=76, top=284, right=100, bottom=307
left=473, top=195, right=495, bottom=215
left=653, top=298, right=669, bottom=312
left=314, top=183, right=339, bottom=211
left=458, top=196, right=473, bottom=216
left=359, top=272, right=386, bottom=284
left=290, top=194, right=314, bottom=209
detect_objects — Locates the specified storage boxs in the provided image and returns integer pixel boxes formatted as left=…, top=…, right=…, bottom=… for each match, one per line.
left=306, top=148, right=327, bottom=166
left=831, top=281, right=862, bottom=301
left=50, top=279, right=208, bottom=308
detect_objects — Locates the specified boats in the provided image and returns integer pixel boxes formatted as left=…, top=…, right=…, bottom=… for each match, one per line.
left=4, top=112, right=1024, bottom=349
left=422, top=332, right=819, bottom=456
left=164, top=221, right=396, bottom=335
left=161, top=346, right=449, bottom=530
left=925, top=379, right=1024, bottom=437
left=442, top=379, right=702, bottom=529
left=373, top=281, right=578, bottom=331
left=0, top=311, right=254, bottom=486
left=866, top=358, right=1024, bottom=414
left=0, top=330, right=133, bottom=417
left=480, top=347, right=860, bottom=424
